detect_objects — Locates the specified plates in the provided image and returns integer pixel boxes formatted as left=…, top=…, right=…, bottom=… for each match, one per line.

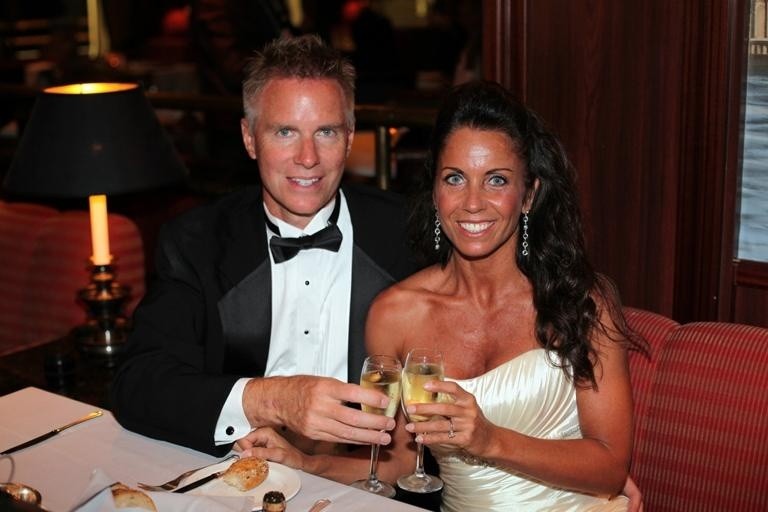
left=186, top=461, right=302, bottom=511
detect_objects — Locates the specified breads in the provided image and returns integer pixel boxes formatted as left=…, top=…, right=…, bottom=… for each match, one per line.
left=113, top=488, right=157, bottom=512
left=225, top=457, right=269, bottom=491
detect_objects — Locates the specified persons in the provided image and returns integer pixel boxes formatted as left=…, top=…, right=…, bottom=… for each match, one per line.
left=117, top=36, right=641, bottom=512
left=232, top=78, right=635, bottom=512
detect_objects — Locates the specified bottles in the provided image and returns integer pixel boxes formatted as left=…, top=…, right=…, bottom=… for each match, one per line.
left=263, top=491, right=288, bottom=511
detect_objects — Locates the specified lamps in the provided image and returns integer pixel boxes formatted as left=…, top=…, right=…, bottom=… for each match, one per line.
left=4, top=80, right=189, bottom=354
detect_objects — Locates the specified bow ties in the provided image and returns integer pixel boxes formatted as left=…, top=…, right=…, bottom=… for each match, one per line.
left=270, top=224, right=343, bottom=264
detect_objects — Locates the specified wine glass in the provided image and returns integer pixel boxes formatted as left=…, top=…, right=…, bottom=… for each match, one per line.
left=350, top=347, right=443, bottom=496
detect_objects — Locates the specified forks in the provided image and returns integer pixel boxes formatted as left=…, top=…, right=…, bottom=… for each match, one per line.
left=138, top=454, right=240, bottom=491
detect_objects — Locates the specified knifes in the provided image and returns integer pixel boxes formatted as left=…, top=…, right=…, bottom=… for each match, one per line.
left=0, top=411, right=102, bottom=455
left=170, top=470, right=228, bottom=494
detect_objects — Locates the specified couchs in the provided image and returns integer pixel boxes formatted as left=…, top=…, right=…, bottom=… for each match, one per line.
left=1, top=201, right=145, bottom=350
left=608, top=305, right=768, bottom=512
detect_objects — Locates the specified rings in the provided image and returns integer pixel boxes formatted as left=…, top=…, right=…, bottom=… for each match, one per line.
left=448, top=419, right=455, bottom=438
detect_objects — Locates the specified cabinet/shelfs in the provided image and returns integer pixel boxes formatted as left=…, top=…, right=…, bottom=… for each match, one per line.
left=0, top=336, right=120, bottom=414
